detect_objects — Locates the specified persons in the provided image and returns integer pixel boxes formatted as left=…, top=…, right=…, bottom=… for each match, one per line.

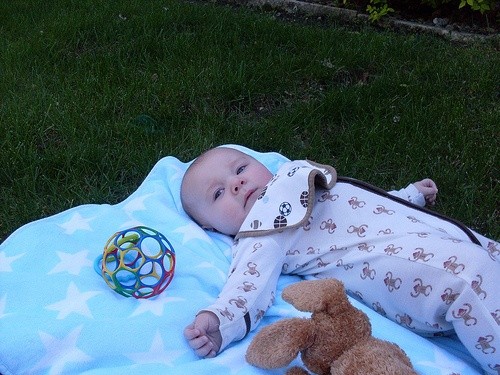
left=180, top=147, right=500, bottom=375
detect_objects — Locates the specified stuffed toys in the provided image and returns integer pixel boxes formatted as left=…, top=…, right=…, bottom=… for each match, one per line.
left=246, top=278, right=417, bottom=375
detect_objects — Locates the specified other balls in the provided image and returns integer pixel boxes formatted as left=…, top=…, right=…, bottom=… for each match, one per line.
left=102, top=226, right=176, bottom=299
